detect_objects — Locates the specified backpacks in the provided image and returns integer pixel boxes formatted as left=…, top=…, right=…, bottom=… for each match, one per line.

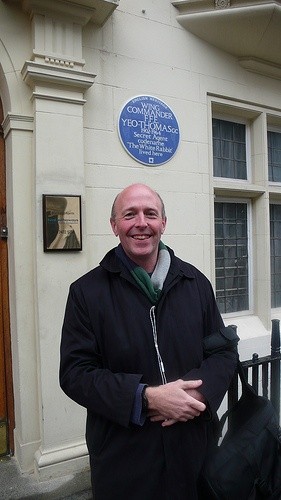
left=196, top=348, right=280, bottom=500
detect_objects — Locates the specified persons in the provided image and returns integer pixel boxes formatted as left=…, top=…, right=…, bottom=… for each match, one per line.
left=59, top=183, right=240, bottom=500
